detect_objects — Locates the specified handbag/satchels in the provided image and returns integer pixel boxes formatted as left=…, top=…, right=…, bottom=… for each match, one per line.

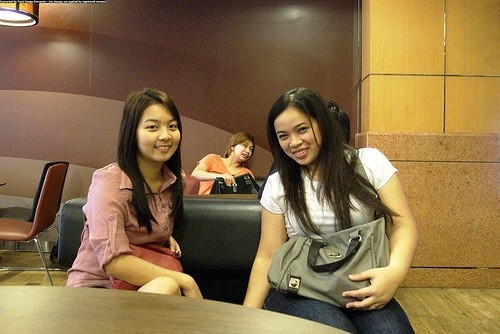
left=109, top=242, right=183, bottom=292
left=209, top=172, right=262, bottom=194
left=267, top=215, right=390, bottom=308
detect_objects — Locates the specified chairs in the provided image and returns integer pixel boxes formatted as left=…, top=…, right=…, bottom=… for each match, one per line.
left=0, top=160, right=69, bottom=285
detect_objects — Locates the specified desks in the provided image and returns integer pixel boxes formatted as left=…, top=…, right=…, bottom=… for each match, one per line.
left=0, top=285, right=350, bottom=334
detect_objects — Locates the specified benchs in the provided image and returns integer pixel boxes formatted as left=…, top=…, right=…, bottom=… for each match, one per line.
left=58, top=178, right=299, bottom=306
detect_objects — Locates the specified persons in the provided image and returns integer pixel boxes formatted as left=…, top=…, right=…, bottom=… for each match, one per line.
left=66, top=87, right=203, bottom=300
left=191, top=131, right=255, bottom=195
left=256, top=99, right=349, bottom=200
left=241, top=87, right=417, bottom=334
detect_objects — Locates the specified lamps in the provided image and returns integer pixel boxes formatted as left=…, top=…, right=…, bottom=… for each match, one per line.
left=0, top=0, right=40, bottom=27
